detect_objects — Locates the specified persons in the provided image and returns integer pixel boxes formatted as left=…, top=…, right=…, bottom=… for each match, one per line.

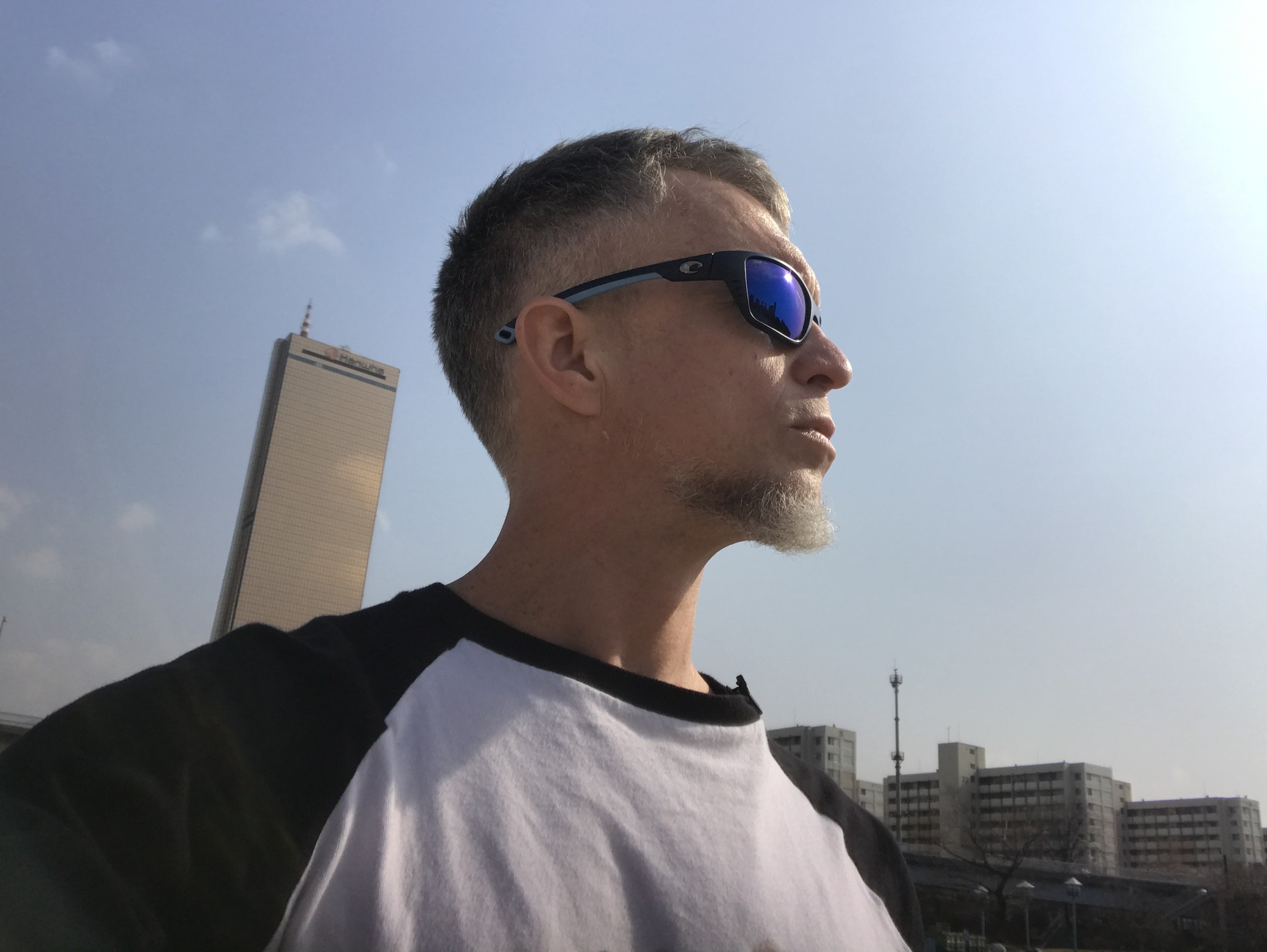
left=0, top=124, right=932, bottom=952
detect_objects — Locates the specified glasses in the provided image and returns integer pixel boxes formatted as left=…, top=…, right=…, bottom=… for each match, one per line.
left=495, top=250, right=821, bottom=350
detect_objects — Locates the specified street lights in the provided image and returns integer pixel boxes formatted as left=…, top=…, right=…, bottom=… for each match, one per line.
left=973, top=885, right=989, bottom=938
left=1063, top=876, right=1083, bottom=950
left=1015, top=880, right=1035, bottom=948
